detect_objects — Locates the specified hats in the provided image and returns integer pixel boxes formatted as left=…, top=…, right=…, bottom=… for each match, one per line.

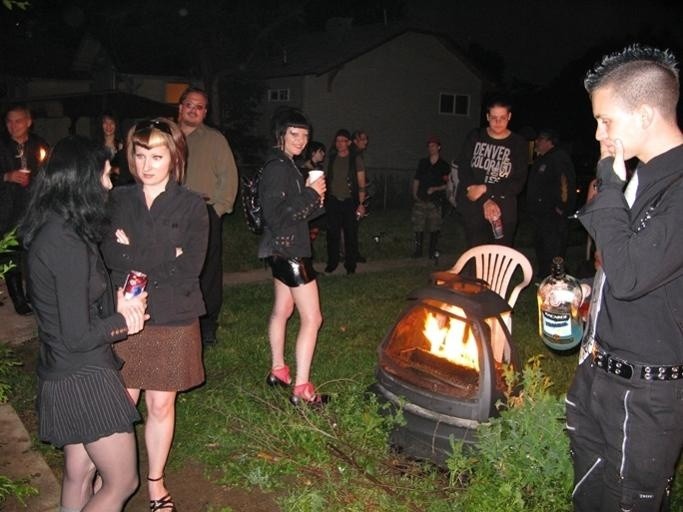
left=427, top=135, right=440, bottom=146
left=336, top=130, right=349, bottom=139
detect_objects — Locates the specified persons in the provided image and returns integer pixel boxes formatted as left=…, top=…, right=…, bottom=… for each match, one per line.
left=95, top=114, right=136, bottom=186
left=350, top=129, right=369, bottom=151
left=456, top=95, right=528, bottom=279
left=15, top=135, right=152, bottom=512
left=538, top=46, right=682, bottom=512
left=293, top=141, right=325, bottom=277
left=176, top=87, right=239, bottom=349
left=410, top=136, right=451, bottom=264
left=0, top=105, right=49, bottom=315
left=94, top=118, right=210, bottom=512
left=250, top=111, right=332, bottom=407
left=525, top=130, right=575, bottom=267
left=326, top=129, right=366, bottom=276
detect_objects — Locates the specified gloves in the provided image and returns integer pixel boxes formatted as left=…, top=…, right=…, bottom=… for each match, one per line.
left=595, top=157, right=626, bottom=191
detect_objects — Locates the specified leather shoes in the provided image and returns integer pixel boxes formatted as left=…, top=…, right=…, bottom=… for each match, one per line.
left=325, top=263, right=338, bottom=273
left=359, top=254, right=368, bottom=263
left=346, top=264, right=357, bottom=273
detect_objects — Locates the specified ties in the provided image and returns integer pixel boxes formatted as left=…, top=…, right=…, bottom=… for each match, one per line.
left=578, top=168, right=640, bottom=366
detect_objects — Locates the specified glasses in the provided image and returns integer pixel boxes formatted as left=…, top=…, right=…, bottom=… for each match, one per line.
left=181, top=101, right=205, bottom=111
left=135, top=119, right=174, bottom=136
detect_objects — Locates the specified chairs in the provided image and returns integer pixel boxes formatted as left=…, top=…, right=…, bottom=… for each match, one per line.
left=446, top=245, right=533, bottom=370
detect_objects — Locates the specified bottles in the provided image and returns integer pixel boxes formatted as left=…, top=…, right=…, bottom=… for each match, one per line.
left=122, top=270, right=148, bottom=300
left=536, top=256, right=584, bottom=357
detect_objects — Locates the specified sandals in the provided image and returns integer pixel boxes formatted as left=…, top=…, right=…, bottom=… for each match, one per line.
left=146, top=472, right=176, bottom=512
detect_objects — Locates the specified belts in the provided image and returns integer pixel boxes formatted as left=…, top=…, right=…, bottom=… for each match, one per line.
left=591, top=352, right=682, bottom=381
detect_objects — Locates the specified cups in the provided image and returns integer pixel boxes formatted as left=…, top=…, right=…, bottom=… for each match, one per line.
left=309, top=170, right=325, bottom=185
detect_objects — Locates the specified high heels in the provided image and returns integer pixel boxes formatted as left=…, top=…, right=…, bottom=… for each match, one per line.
left=290, top=391, right=332, bottom=407
left=339, top=249, right=346, bottom=263
left=267, top=372, right=295, bottom=390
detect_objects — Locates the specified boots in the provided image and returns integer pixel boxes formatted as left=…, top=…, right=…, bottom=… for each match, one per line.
left=3, top=270, right=35, bottom=316
left=431, top=231, right=442, bottom=260
left=411, top=231, right=424, bottom=259
left=24, top=251, right=31, bottom=302
